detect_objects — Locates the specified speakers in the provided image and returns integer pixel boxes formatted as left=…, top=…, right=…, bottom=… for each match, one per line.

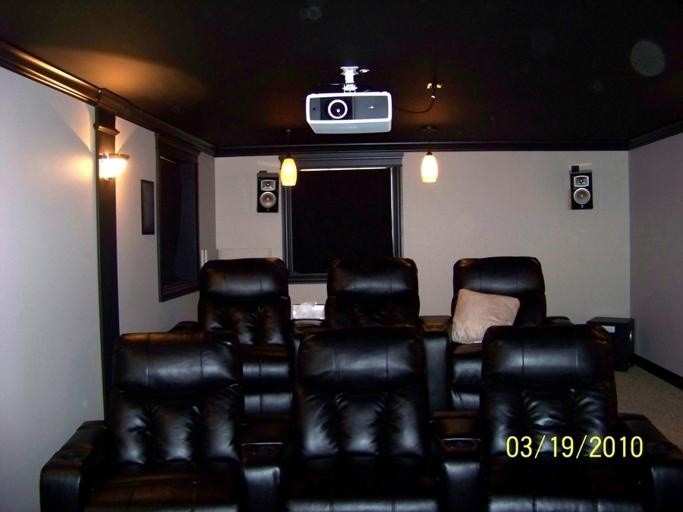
left=257, top=173, right=279, bottom=212
left=587, top=317, right=634, bottom=372
left=569, top=170, right=593, bottom=210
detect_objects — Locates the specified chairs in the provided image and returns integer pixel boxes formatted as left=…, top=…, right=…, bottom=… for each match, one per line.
left=240, top=332, right=442, bottom=512
left=424, top=257, right=573, bottom=415
left=166, top=257, right=295, bottom=421
left=320, top=255, right=421, bottom=329
left=442, top=325, right=682, bottom=512
left=40, top=330, right=240, bottom=512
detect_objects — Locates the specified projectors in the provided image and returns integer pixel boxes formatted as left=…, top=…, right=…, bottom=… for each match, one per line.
left=305, top=91, right=393, bottom=134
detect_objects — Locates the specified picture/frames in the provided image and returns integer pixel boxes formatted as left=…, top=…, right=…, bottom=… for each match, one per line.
left=141, top=180, right=154, bottom=235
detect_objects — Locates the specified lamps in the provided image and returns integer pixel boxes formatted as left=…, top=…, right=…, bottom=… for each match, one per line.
left=420, top=125, right=438, bottom=183
left=279, top=127, right=298, bottom=186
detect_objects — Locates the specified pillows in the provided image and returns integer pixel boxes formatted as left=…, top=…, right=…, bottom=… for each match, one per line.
left=450, top=288, right=520, bottom=345
